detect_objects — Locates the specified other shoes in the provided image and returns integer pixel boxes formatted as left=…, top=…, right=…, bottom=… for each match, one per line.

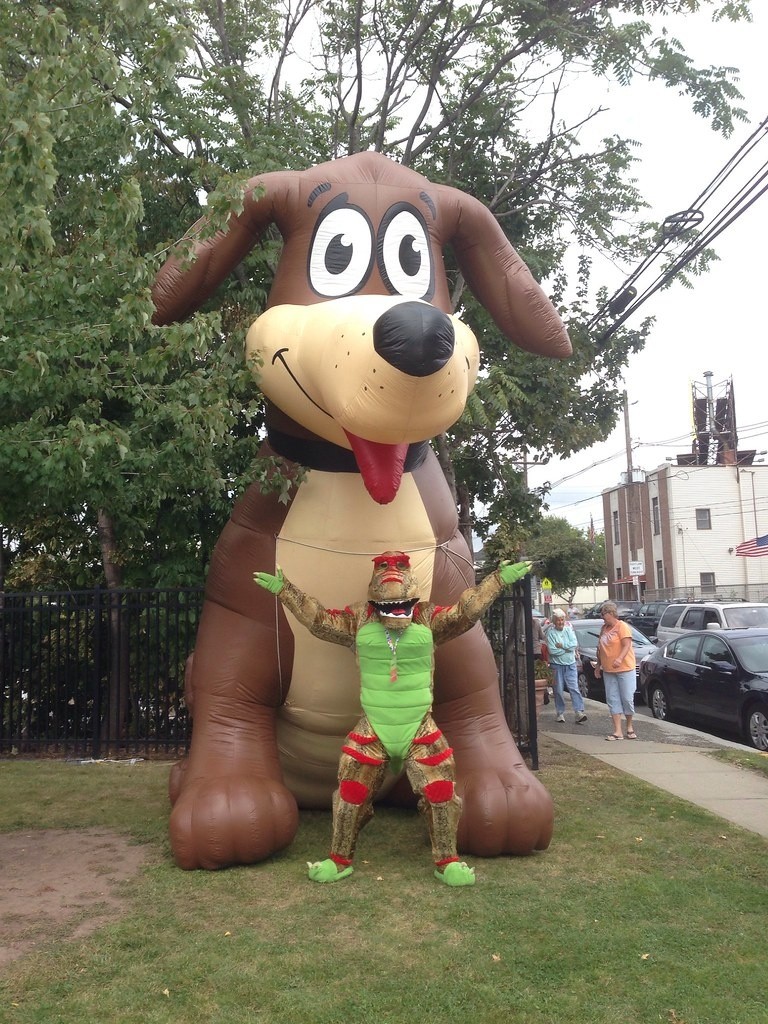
left=433, top=861, right=476, bottom=887
left=306, top=859, right=353, bottom=882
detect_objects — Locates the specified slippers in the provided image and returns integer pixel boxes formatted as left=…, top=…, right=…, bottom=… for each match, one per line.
left=626, top=732, right=636, bottom=739
left=604, top=734, right=623, bottom=741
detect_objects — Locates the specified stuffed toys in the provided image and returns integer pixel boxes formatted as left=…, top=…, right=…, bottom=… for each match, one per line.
left=252, top=549, right=536, bottom=887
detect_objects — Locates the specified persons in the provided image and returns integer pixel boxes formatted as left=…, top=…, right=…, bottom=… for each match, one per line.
left=593, top=600, right=638, bottom=742
left=532, top=617, right=551, bottom=705
left=539, top=617, right=552, bottom=670
left=566, top=602, right=581, bottom=620
left=544, top=608, right=588, bottom=724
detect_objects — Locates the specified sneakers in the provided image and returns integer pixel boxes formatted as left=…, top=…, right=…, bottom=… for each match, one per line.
left=574, top=713, right=587, bottom=722
left=556, top=714, right=565, bottom=722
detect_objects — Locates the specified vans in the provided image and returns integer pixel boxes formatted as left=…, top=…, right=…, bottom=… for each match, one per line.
left=656, top=603, right=768, bottom=647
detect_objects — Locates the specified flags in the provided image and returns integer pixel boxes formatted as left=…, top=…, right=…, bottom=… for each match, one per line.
left=735, top=534, right=768, bottom=558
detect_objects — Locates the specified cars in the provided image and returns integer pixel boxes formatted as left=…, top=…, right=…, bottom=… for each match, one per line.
left=640, top=627, right=768, bottom=751
left=624, top=603, right=668, bottom=635
left=533, top=600, right=643, bottom=626
left=570, top=619, right=657, bottom=699
